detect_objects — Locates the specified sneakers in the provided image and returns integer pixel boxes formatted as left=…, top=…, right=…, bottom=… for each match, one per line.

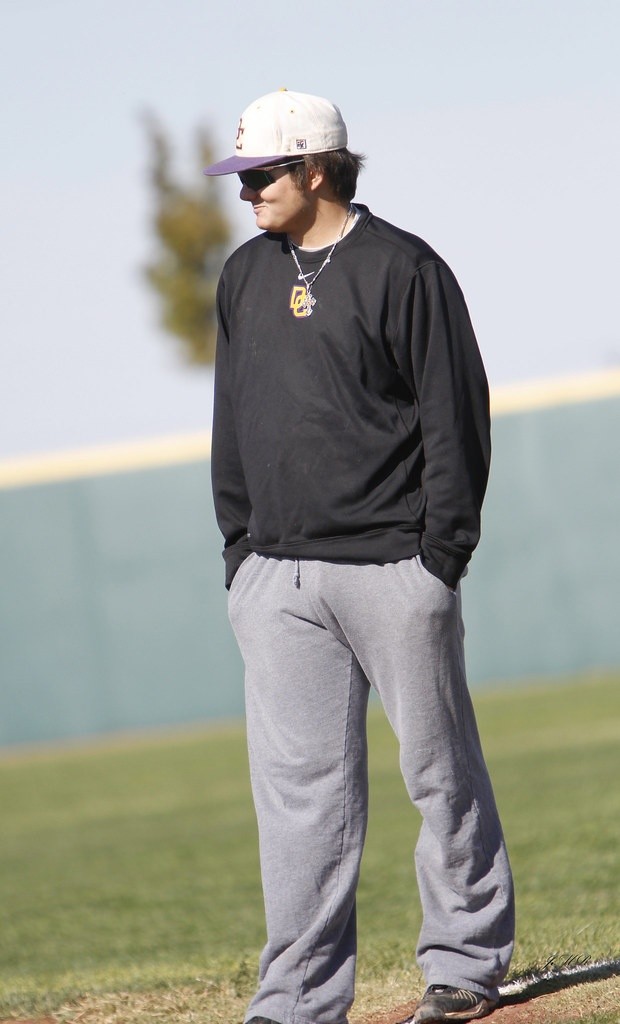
left=413, top=984, right=496, bottom=1024
left=243, top=1015, right=280, bottom=1024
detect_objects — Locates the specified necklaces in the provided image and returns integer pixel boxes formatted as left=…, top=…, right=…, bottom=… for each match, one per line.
left=287, top=203, right=355, bottom=319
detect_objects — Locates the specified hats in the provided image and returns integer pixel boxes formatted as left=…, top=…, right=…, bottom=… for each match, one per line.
left=201, top=88, right=347, bottom=175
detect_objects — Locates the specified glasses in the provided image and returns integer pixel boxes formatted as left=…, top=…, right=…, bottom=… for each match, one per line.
left=238, top=159, right=306, bottom=191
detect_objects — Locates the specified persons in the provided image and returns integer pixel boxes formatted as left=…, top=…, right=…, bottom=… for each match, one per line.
left=205, top=88, right=517, bottom=1024
